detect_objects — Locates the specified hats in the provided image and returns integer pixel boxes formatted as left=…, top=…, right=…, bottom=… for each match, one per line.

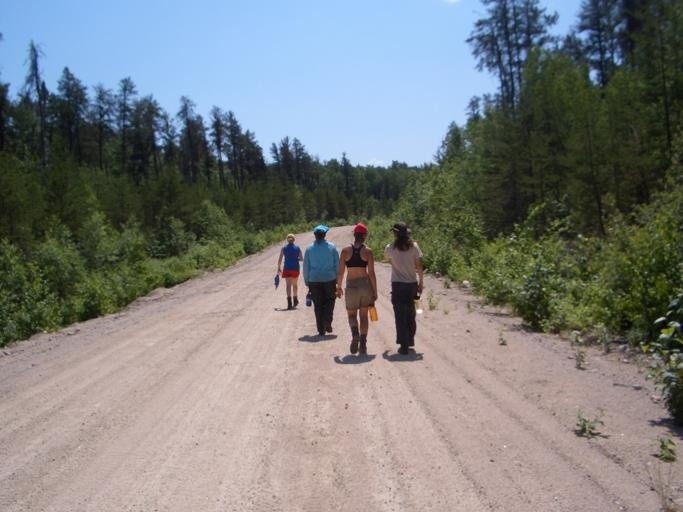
left=354, top=224, right=367, bottom=233
left=315, top=225, right=329, bottom=233
left=390, top=223, right=406, bottom=232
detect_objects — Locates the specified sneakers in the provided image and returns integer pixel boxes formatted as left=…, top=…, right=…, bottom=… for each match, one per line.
left=319, top=320, right=332, bottom=335
left=398, top=337, right=414, bottom=355
left=350, top=336, right=366, bottom=353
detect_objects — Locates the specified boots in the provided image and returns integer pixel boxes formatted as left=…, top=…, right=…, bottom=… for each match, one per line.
left=288, top=296, right=298, bottom=309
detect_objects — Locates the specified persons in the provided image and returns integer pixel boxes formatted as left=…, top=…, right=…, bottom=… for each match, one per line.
left=336, top=223, right=378, bottom=355
left=277, top=234, right=304, bottom=309
left=302, top=224, right=340, bottom=336
left=383, top=221, right=424, bottom=355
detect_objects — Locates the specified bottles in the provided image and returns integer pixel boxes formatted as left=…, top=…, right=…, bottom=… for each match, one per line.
left=305, top=293, right=312, bottom=307
left=414, top=295, right=424, bottom=313
left=368, top=304, right=379, bottom=321
